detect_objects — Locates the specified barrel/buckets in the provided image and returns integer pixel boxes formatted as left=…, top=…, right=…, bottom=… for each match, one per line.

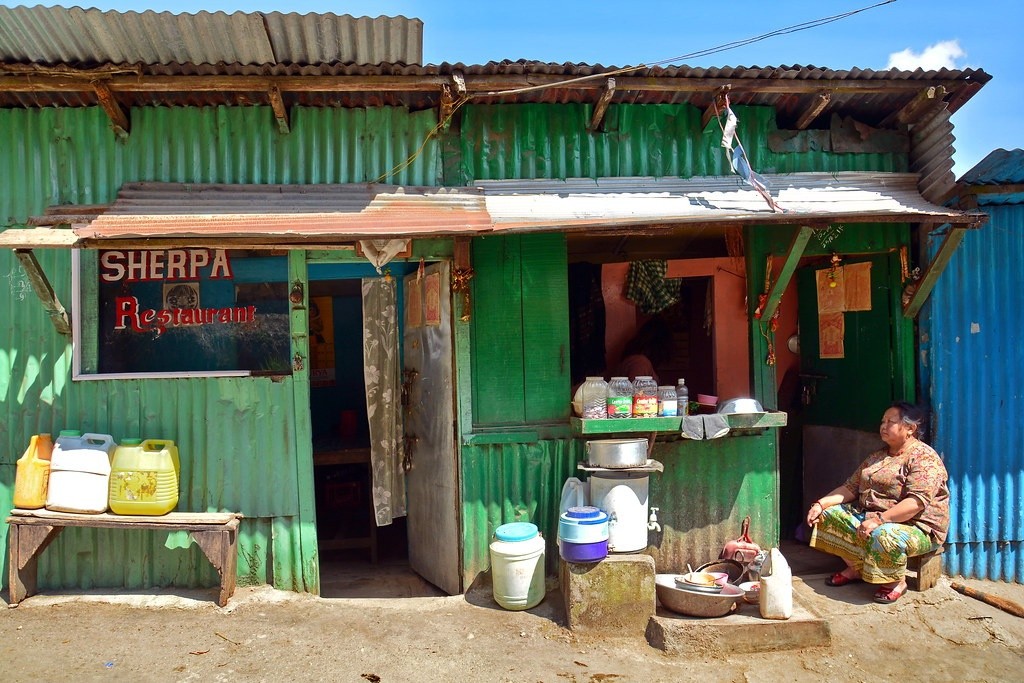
left=559, top=506, right=608, bottom=563
left=109, top=438, right=179, bottom=516
left=758, top=548, right=792, bottom=620
left=490, top=521, right=546, bottom=610
left=46, top=430, right=117, bottom=515
left=590, top=471, right=649, bottom=554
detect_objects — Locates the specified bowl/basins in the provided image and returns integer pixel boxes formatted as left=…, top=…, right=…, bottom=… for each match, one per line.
left=698, top=394, right=719, bottom=405
left=656, top=571, right=746, bottom=617
left=571, top=402, right=582, bottom=418
left=714, top=398, right=766, bottom=416
left=585, top=438, right=649, bottom=468
left=738, top=581, right=760, bottom=604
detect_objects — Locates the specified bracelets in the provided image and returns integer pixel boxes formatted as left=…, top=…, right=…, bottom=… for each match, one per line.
left=872, top=509, right=887, bottom=525
left=812, top=499, right=822, bottom=513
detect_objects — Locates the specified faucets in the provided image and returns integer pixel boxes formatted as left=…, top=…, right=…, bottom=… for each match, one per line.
left=647, top=507, right=661, bottom=532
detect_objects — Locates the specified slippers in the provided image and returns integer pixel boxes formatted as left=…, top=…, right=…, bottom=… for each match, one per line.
left=873, top=585, right=907, bottom=604
left=825, top=572, right=863, bottom=587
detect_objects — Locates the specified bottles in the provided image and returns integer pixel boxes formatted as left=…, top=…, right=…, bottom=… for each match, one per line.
left=632, top=376, right=657, bottom=418
left=678, top=378, right=689, bottom=416
left=658, top=386, right=678, bottom=416
left=608, top=377, right=633, bottom=419
left=14, top=433, right=53, bottom=509
left=582, top=377, right=608, bottom=420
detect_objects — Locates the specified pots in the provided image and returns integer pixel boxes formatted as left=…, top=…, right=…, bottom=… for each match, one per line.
left=694, top=550, right=745, bottom=586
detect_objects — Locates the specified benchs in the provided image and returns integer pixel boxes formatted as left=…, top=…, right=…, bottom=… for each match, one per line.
left=3, top=511, right=242, bottom=608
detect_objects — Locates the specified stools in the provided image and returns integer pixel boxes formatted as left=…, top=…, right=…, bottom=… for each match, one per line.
left=908, top=547, right=944, bottom=591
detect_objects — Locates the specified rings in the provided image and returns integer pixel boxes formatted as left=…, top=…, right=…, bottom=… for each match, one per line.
left=857, top=529, right=866, bottom=535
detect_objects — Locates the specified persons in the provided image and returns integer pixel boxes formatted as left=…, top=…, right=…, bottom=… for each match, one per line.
left=616, top=325, right=671, bottom=392
left=806, top=402, right=951, bottom=605
